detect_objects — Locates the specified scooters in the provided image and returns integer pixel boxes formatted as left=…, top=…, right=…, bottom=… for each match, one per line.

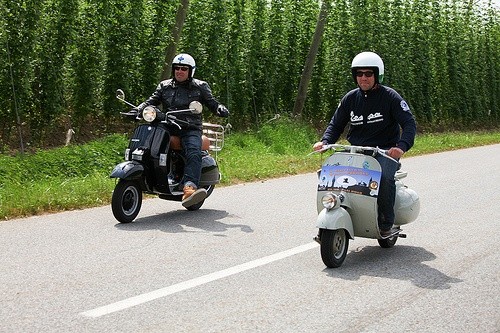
left=304, top=144, right=419, bottom=269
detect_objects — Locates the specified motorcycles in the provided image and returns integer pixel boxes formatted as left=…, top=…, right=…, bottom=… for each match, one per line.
left=109, top=89, right=232, bottom=224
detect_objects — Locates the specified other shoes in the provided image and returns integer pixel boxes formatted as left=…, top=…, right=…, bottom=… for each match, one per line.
left=180, top=185, right=208, bottom=207
left=381, top=227, right=393, bottom=236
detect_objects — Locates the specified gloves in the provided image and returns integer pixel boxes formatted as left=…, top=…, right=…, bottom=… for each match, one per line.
left=217, top=105, right=231, bottom=118
left=124, top=110, right=139, bottom=122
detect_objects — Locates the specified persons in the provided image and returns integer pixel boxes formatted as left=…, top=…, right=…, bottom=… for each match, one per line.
left=313, top=52, right=415, bottom=245
left=127, top=53, right=229, bottom=207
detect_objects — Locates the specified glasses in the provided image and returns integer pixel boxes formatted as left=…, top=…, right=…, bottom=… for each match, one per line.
left=356, top=71, right=374, bottom=77
left=174, top=66, right=189, bottom=71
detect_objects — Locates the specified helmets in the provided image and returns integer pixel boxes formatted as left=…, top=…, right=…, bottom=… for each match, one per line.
left=171, top=53, right=196, bottom=82
left=351, top=51, right=385, bottom=85
